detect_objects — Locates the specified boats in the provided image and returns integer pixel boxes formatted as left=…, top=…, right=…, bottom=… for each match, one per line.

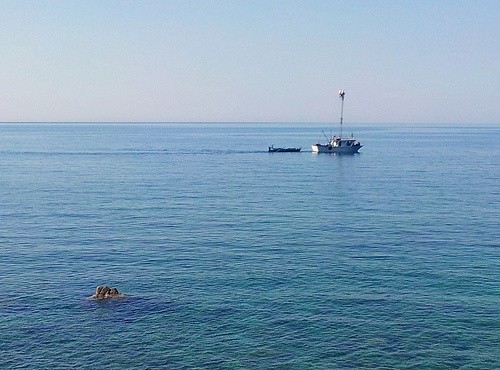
left=269, top=144, right=301, bottom=152
left=311, top=131, right=364, bottom=154
